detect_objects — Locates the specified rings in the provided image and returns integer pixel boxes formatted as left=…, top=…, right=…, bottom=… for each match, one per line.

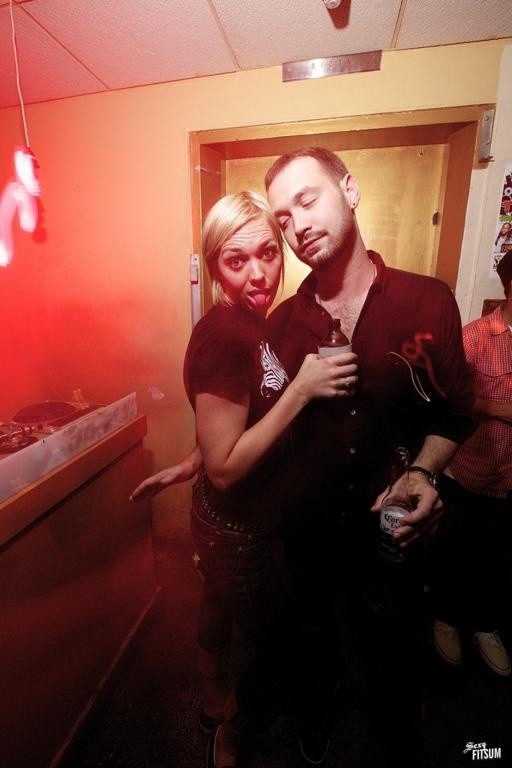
left=343, top=375, right=350, bottom=388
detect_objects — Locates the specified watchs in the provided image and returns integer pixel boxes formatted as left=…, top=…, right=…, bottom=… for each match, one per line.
left=407, top=463, right=440, bottom=490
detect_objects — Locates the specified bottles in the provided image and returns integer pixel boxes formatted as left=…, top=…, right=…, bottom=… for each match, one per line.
left=378, top=446, right=414, bottom=561
left=315, top=319, right=352, bottom=411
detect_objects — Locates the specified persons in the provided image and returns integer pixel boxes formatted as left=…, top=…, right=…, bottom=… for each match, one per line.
left=131, top=149, right=474, bottom=767
left=424, top=247, right=510, bottom=674
left=493, top=222, right=512, bottom=255
left=187, top=191, right=367, bottom=762
